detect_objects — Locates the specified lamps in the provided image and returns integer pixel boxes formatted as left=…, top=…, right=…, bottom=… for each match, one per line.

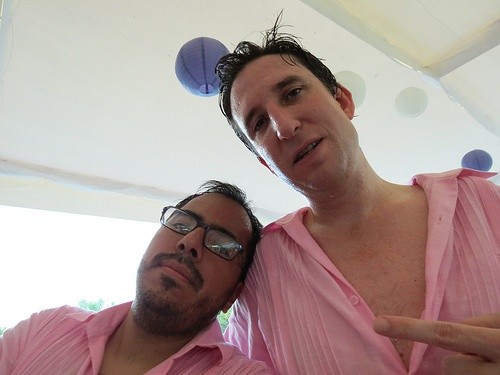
left=460, top=148, right=493, bottom=172
left=175, top=37, right=232, bottom=96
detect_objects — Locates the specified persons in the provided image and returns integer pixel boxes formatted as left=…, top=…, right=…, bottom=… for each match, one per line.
left=214, top=9, right=500, bottom=375
left=0, top=181, right=277, bottom=375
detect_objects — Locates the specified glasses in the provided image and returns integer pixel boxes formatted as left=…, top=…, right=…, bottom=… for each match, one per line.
left=156, top=203, right=248, bottom=286
